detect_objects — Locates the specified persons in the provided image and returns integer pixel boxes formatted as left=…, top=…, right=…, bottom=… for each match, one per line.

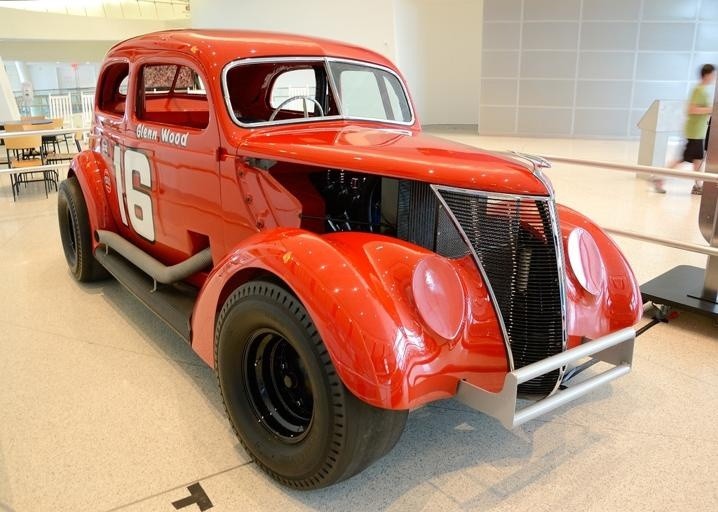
left=653, top=63, right=716, bottom=194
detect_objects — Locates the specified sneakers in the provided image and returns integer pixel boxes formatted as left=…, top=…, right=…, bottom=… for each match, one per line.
left=652, top=177, right=667, bottom=195
left=691, top=186, right=704, bottom=195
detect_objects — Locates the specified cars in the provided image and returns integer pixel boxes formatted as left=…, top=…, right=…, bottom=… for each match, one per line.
left=58, top=29, right=643, bottom=491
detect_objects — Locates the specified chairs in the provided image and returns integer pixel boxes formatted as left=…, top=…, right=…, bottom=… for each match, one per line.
left=3, top=92, right=94, bottom=202
